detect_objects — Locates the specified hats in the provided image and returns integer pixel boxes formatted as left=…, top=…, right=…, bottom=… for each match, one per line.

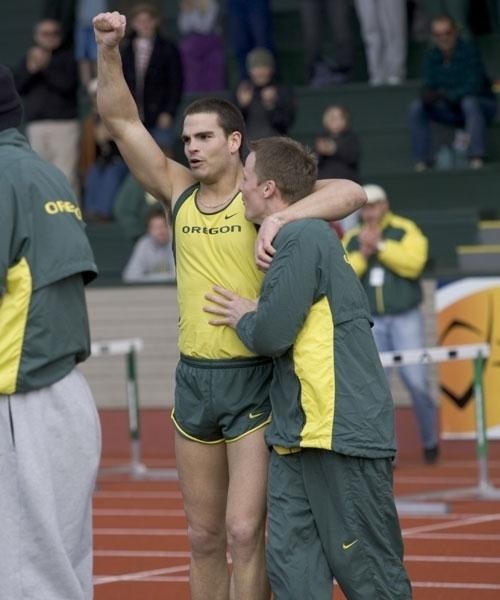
left=357, top=182, right=387, bottom=205
left=0, top=64, right=24, bottom=133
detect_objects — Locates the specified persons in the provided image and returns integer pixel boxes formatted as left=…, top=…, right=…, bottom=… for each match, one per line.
left=92, top=11, right=368, bottom=599
left=337, top=183, right=441, bottom=466
left=1, top=1, right=499, bottom=285
left=201, top=135, right=412, bottom=600
left=0, top=64, right=102, bottom=600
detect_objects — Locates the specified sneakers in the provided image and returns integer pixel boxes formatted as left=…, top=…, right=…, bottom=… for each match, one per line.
left=423, top=443, right=440, bottom=465
